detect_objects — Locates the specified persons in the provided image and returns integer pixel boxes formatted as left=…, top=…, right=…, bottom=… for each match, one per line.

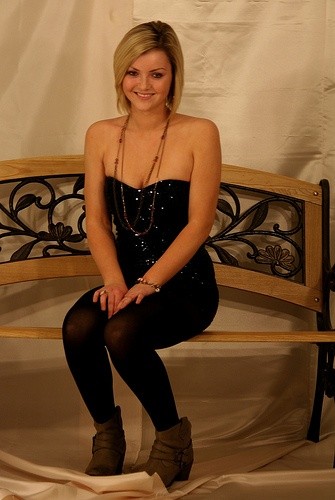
left=62, top=20, right=223, bottom=489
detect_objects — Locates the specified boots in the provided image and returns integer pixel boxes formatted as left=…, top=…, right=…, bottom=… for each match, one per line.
left=144, top=416, right=195, bottom=488
left=83, top=406, right=128, bottom=476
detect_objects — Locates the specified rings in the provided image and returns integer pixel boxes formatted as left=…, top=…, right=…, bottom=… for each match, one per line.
left=99, top=290, right=107, bottom=295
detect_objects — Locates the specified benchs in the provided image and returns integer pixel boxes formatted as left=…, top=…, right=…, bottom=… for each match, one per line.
left=0, top=156, right=335, bottom=443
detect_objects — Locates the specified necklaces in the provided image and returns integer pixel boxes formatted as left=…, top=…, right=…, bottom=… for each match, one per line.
left=113, top=112, right=172, bottom=238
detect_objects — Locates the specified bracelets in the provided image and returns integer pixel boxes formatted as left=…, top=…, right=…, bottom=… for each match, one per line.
left=136, top=277, right=161, bottom=293
